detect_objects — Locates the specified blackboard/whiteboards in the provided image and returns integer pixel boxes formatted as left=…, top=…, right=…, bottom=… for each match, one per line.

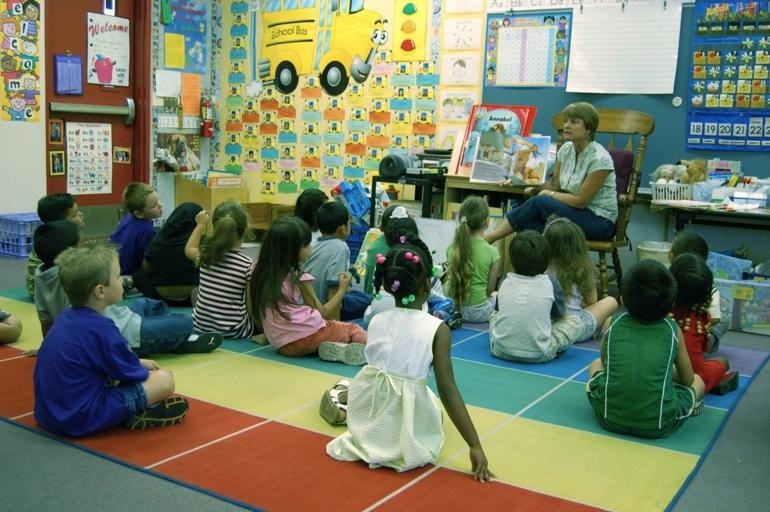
left=481, top=3, right=770, bottom=194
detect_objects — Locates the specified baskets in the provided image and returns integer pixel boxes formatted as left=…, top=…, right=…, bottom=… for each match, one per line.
left=0, top=211, right=45, bottom=260
left=648, top=180, right=693, bottom=202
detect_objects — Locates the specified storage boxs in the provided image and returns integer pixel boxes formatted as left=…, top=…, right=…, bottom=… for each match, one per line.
left=175, top=173, right=244, bottom=213
left=717, top=278, right=770, bottom=340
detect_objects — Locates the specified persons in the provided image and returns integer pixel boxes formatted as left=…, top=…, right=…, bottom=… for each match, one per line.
left=485, top=102, right=620, bottom=251
left=1, top=308, right=23, bottom=344
left=443, top=196, right=617, bottom=364
left=584, top=231, right=740, bottom=438
left=250, top=187, right=496, bottom=483
left=27, top=183, right=266, bottom=439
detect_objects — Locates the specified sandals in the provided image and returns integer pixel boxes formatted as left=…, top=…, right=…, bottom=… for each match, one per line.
left=320, top=378, right=351, bottom=426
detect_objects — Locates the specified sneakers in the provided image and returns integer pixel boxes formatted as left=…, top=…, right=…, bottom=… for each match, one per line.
left=123, top=394, right=190, bottom=432
left=446, top=311, right=463, bottom=330
left=316, top=339, right=369, bottom=368
left=690, top=398, right=705, bottom=417
left=713, top=370, right=739, bottom=396
left=161, top=331, right=225, bottom=357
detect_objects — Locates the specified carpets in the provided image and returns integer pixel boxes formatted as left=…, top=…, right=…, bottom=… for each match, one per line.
left=0, top=273, right=770, bottom=510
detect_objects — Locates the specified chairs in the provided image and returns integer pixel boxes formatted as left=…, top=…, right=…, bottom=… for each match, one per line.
left=551, top=107, right=658, bottom=296
left=240, top=201, right=294, bottom=232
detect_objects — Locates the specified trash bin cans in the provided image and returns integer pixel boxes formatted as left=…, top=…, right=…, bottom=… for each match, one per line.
left=638, top=241, right=672, bottom=263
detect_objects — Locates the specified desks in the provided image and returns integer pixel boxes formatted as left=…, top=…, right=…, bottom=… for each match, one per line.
left=442, top=175, right=525, bottom=273
left=667, top=205, right=770, bottom=242
left=369, top=176, right=430, bottom=229
